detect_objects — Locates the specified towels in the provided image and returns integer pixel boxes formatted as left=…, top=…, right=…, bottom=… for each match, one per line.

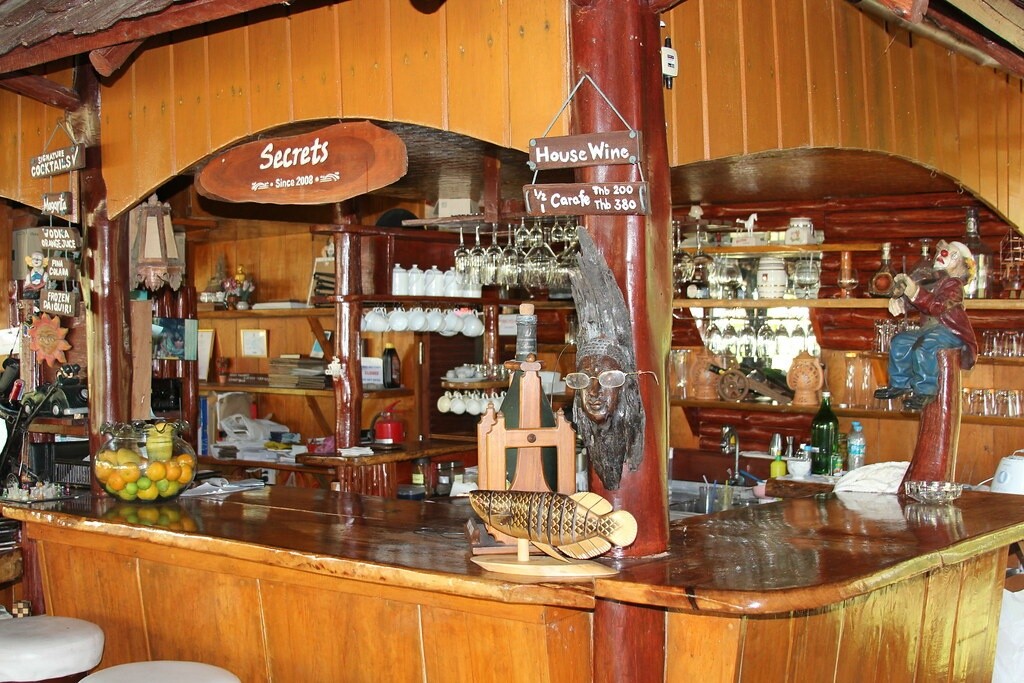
left=833, top=460, right=910, bottom=495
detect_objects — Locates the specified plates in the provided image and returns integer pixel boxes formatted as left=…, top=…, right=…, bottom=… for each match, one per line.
left=439, top=377, right=487, bottom=383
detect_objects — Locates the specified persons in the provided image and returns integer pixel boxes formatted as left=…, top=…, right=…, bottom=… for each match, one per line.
left=874, top=240, right=978, bottom=409
left=23, top=253, right=49, bottom=299
left=564, top=337, right=639, bottom=487
left=203, top=260, right=254, bottom=302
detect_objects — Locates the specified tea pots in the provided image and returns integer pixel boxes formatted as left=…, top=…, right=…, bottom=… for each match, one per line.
left=454, top=363, right=476, bottom=378
left=391, top=262, right=482, bottom=299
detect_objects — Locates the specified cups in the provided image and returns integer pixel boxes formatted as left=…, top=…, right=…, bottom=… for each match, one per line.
left=980, top=329, right=1024, bottom=357
left=665, top=349, right=696, bottom=400
left=436, top=390, right=508, bottom=415
left=698, top=486, right=735, bottom=513
left=358, top=305, right=486, bottom=337
left=872, top=318, right=921, bottom=357
left=445, top=365, right=508, bottom=381
left=959, top=387, right=1024, bottom=417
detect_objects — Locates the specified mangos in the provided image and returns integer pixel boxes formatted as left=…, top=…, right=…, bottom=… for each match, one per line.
left=95, top=448, right=140, bottom=481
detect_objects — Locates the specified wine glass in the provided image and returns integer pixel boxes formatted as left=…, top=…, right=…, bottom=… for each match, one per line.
left=666, top=218, right=717, bottom=288
left=794, top=261, right=820, bottom=300
left=450, top=213, right=581, bottom=286
left=837, top=267, right=859, bottom=299
left=704, top=315, right=819, bottom=355
left=716, top=259, right=743, bottom=301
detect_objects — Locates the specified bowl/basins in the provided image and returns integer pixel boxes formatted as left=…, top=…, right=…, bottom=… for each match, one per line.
left=787, top=458, right=810, bottom=476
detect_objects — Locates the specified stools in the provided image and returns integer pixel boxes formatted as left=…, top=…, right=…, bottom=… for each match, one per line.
left=0, top=616, right=104, bottom=683
left=77, top=660, right=242, bottom=683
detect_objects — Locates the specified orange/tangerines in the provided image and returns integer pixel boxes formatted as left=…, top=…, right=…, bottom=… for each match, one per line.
left=105, top=454, right=195, bottom=501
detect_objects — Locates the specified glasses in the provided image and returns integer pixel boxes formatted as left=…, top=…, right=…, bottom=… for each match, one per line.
left=563, top=370, right=660, bottom=390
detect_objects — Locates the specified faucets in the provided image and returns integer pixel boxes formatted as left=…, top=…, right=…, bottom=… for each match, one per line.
left=719, top=430, right=744, bottom=485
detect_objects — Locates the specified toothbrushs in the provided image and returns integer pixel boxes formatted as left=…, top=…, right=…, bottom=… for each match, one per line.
left=703, top=475, right=717, bottom=514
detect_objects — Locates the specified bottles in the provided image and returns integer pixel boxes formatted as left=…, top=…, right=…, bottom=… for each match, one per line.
left=846, top=421, right=866, bottom=472
left=867, top=242, right=899, bottom=299
left=960, top=206, right=995, bottom=300
left=93, top=419, right=197, bottom=502
left=810, top=391, right=840, bottom=475
left=843, top=351, right=876, bottom=410
left=907, top=239, right=938, bottom=293
left=754, top=258, right=788, bottom=299
left=769, top=451, right=787, bottom=478
left=784, top=217, right=818, bottom=246
left=408, top=457, right=464, bottom=495
left=382, top=343, right=401, bottom=389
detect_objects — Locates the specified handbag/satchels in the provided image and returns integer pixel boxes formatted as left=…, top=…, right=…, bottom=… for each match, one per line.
left=210, top=414, right=290, bottom=460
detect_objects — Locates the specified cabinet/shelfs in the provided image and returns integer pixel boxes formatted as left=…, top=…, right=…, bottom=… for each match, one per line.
left=195, top=308, right=415, bottom=437
left=294, top=203, right=1024, bottom=571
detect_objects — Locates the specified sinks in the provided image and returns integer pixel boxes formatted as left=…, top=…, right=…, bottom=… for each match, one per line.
left=669, top=499, right=733, bottom=514
